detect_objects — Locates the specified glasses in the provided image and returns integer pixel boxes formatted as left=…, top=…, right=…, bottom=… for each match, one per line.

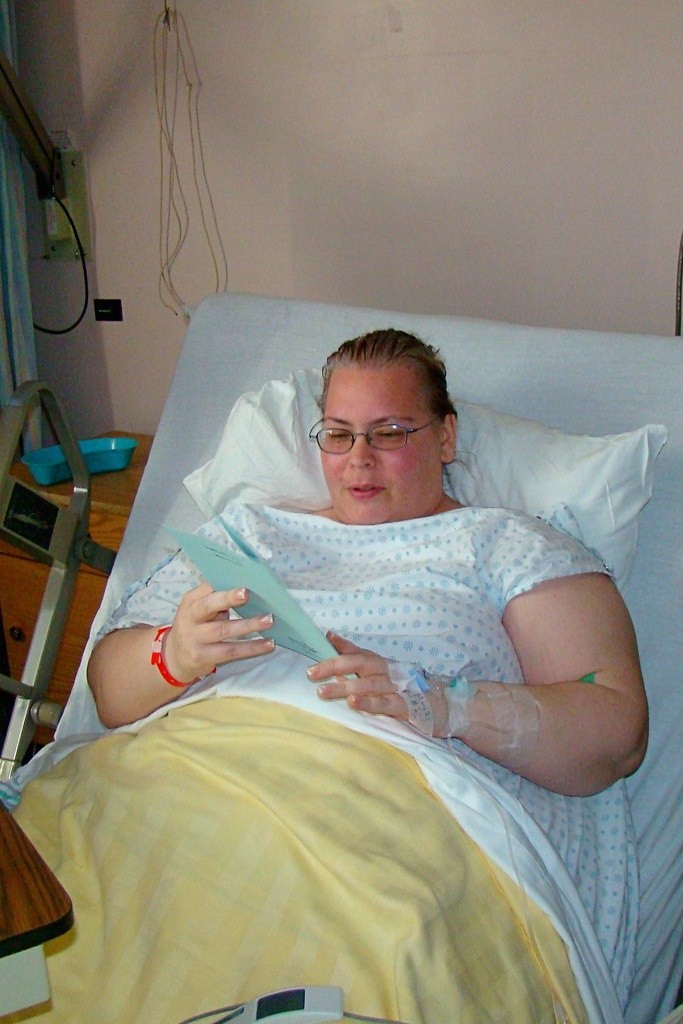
left=308, top=416, right=441, bottom=454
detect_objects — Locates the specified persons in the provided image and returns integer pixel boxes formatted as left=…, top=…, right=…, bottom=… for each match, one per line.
left=86, top=329, right=648, bottom=1017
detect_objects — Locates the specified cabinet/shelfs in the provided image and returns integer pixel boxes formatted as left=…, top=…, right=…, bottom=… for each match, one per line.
left=0, top=431, right=154, bottom=744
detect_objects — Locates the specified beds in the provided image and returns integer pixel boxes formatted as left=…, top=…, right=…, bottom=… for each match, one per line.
left=0, top=292, right=683, bottom=1024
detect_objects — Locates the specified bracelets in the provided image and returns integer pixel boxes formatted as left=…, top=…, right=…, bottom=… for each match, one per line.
left=149, top=624, right=216, bottom=689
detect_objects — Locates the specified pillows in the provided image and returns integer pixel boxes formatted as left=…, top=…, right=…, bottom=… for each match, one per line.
left=182, top=369, right=670, bottom=595
